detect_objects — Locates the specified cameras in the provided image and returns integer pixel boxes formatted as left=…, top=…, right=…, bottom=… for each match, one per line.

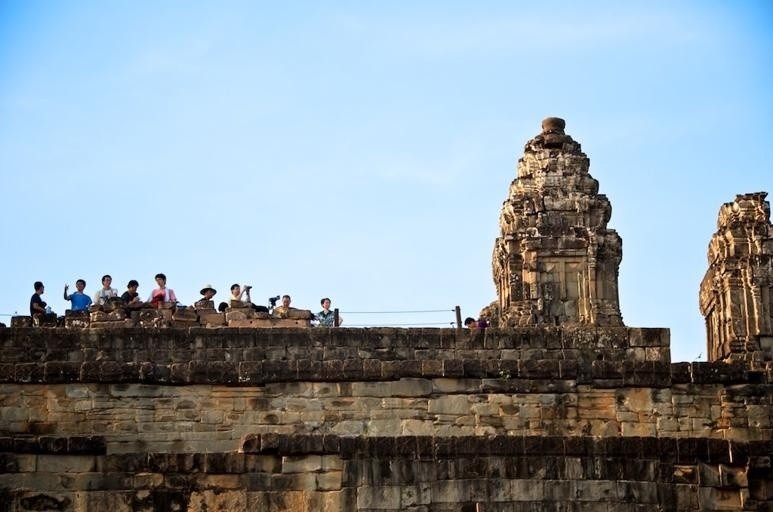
left=269, top=296, right=280, bottom=306
left=244, top=285, right=252, bottom=290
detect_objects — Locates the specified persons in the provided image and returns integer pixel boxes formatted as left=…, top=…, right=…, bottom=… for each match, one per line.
left=464, top=317, right=487, bottom=329
left=145, top=273, right=177, bottom=307
left=278, top=295, right=295, bottom=309
left=64, top=279, right=92, bottom=311
left=192, top=285, right=216, bottom=301
left=92, top=274, right=117, bottom=305
left=219, top=283, right=252, bottom=312
left=121, top=279, right=140, bottom=304
left=312, top=297, right=342, bottom=326
left=30, top=281, right=47, bottom=325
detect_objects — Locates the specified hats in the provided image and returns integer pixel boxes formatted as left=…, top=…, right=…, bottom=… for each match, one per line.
left=199, top=285, right=218, bottom=295
